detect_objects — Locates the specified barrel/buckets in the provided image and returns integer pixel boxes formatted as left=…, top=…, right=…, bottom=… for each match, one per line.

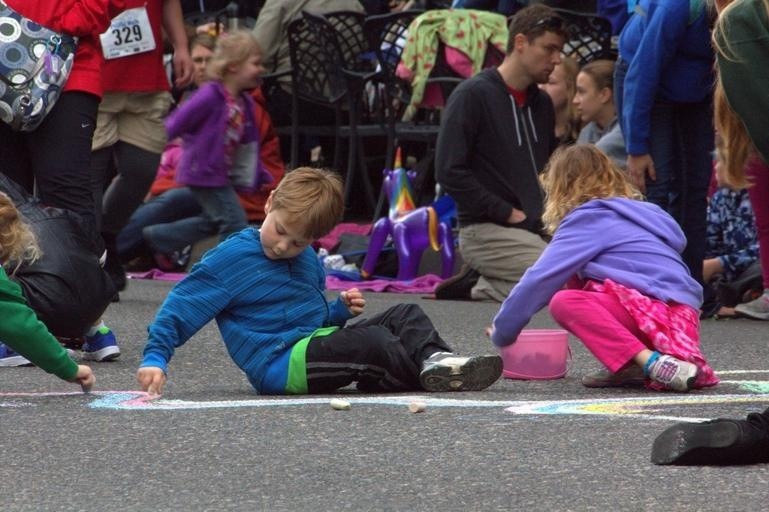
left=501, top=329, right=574, bottom=380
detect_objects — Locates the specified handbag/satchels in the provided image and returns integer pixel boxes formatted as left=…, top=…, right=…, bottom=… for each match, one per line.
left=0, top=0, right=80, bottom=132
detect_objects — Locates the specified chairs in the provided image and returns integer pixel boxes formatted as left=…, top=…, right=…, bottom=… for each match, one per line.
left=507, top=10, right=613, bottom=68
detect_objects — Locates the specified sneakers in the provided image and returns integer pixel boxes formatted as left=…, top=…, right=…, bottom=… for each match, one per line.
left=434, top=263, right=481, bottom=299
left=582, top=365, right=646, bottom=387
left=734, top=299, right=769, bottom=320
left=649, top=355, right=698, bottom=393
left=419, top=352, right=503, bottom=392
left=99, top=230, right=126, bottom=291
left=81, top=327, right=120, bottom=363
left=0, top=342, right=32, bottom=368
left=651, top=419, right=743, bottom=466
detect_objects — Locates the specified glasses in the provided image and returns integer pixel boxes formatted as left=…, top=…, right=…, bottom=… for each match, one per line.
left=535, top=16, right=562, bottom=27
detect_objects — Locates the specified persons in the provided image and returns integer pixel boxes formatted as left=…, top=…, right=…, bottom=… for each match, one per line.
left=487, top=144, right=719, bottom=393
left=2, top=1, right=768, bottom=366
left=650, top=409, right=769, bottom=466
left=137, top=166, right=504, bottom=396
left=0, top=191, right=96, bottom=392
